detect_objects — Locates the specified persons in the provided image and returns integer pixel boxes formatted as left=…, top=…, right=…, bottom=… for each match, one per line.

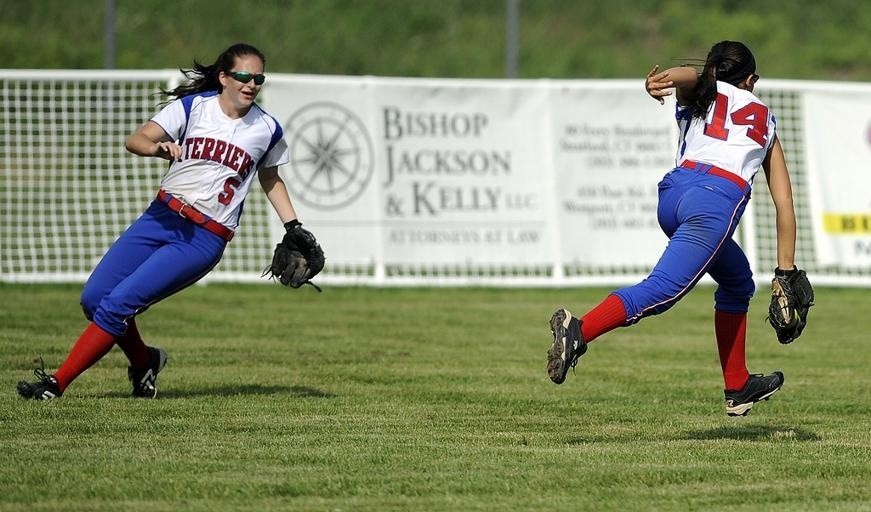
left=16, top=41, right=326, bottom=403
left=546, top=39, right=815, bottom=419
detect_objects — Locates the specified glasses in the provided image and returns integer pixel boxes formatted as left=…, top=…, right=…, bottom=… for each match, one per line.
left=226, top=70, right=266, bottom=86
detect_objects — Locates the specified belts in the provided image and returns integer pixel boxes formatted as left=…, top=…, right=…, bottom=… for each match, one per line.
left=157, top=189, right=234, bottom=244
left=679, top=159, right=751, bottom=195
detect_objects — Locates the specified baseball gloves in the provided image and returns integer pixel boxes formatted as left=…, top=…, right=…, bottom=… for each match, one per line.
left=769, top=265, right=815, bottom=344
left=262, top=227, right=326, bottom=291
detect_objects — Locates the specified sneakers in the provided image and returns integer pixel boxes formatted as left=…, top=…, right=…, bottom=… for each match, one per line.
left=547, top=308, right=588, bottom=385
left=129, top=345, right=167, bottom=401
left=723, top=370, right=785, bottom=416
left=17, top=373, right=62, bottom=401
